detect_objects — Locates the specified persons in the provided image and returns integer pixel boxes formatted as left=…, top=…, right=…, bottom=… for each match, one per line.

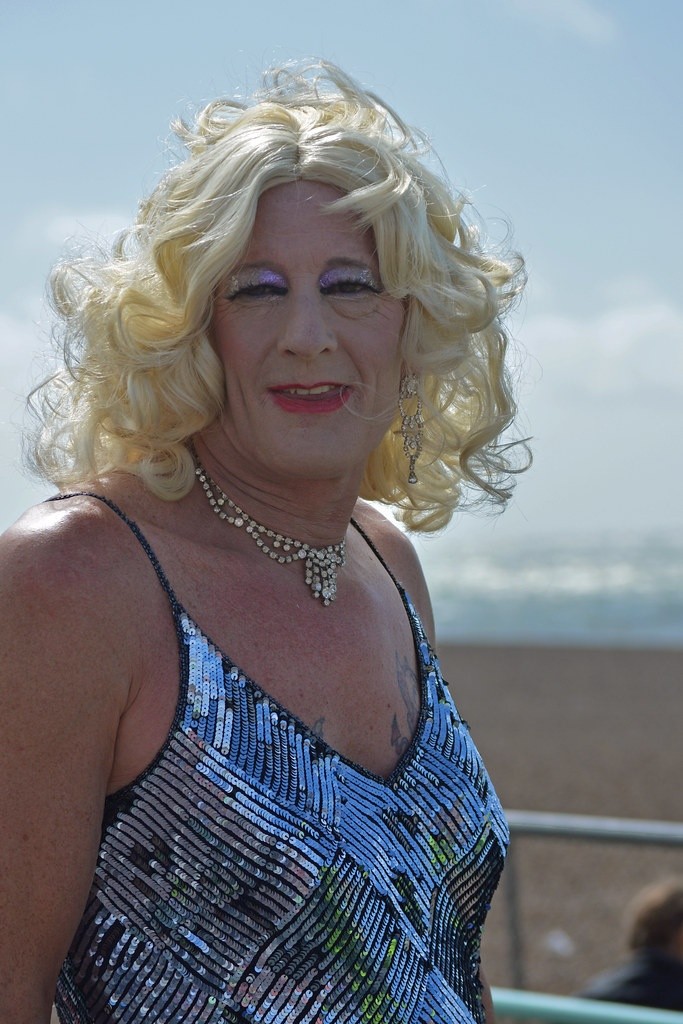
left=0, top=64, right=533, bottom=1024
left=580, top=881, right=683, bottom=1013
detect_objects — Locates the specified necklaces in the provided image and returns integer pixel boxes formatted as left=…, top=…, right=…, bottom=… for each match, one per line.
left=186, top=435, right=347, bottom=607
